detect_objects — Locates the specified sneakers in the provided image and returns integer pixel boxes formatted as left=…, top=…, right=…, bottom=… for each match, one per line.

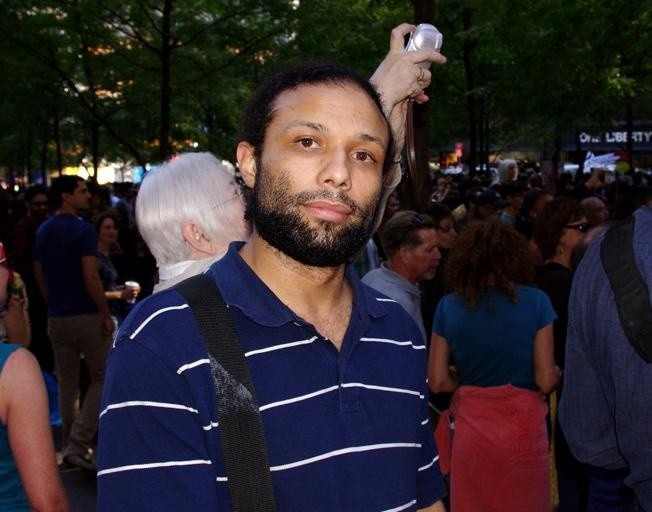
left=58, top=444, right=98, bottom=472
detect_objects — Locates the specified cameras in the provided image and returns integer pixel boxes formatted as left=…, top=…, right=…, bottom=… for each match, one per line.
left=406, top=24, right=443, bottom=69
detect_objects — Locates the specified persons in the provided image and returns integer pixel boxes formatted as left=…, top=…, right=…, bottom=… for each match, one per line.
left=134, top=24, right=447, bottom=296
left=0, top=153, right=250, bottom=511
left=372, top=158, right=650, bottom=511
left=98, top=61, right=449, bottom=512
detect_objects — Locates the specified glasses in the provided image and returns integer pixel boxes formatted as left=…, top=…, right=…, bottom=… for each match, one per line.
left=564, top=220, right=590, bottom=234
left=402, top=211, right=423, bottom=239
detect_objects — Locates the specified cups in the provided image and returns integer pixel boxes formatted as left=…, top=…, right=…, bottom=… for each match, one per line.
left=124, top=281, right=139, bottom=303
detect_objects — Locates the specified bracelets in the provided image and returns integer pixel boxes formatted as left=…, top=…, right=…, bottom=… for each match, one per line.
left=389, top=156, right=404, bottom=165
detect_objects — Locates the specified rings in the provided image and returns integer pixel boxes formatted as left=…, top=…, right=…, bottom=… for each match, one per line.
left=417, top=66, right=426, bottom=81
